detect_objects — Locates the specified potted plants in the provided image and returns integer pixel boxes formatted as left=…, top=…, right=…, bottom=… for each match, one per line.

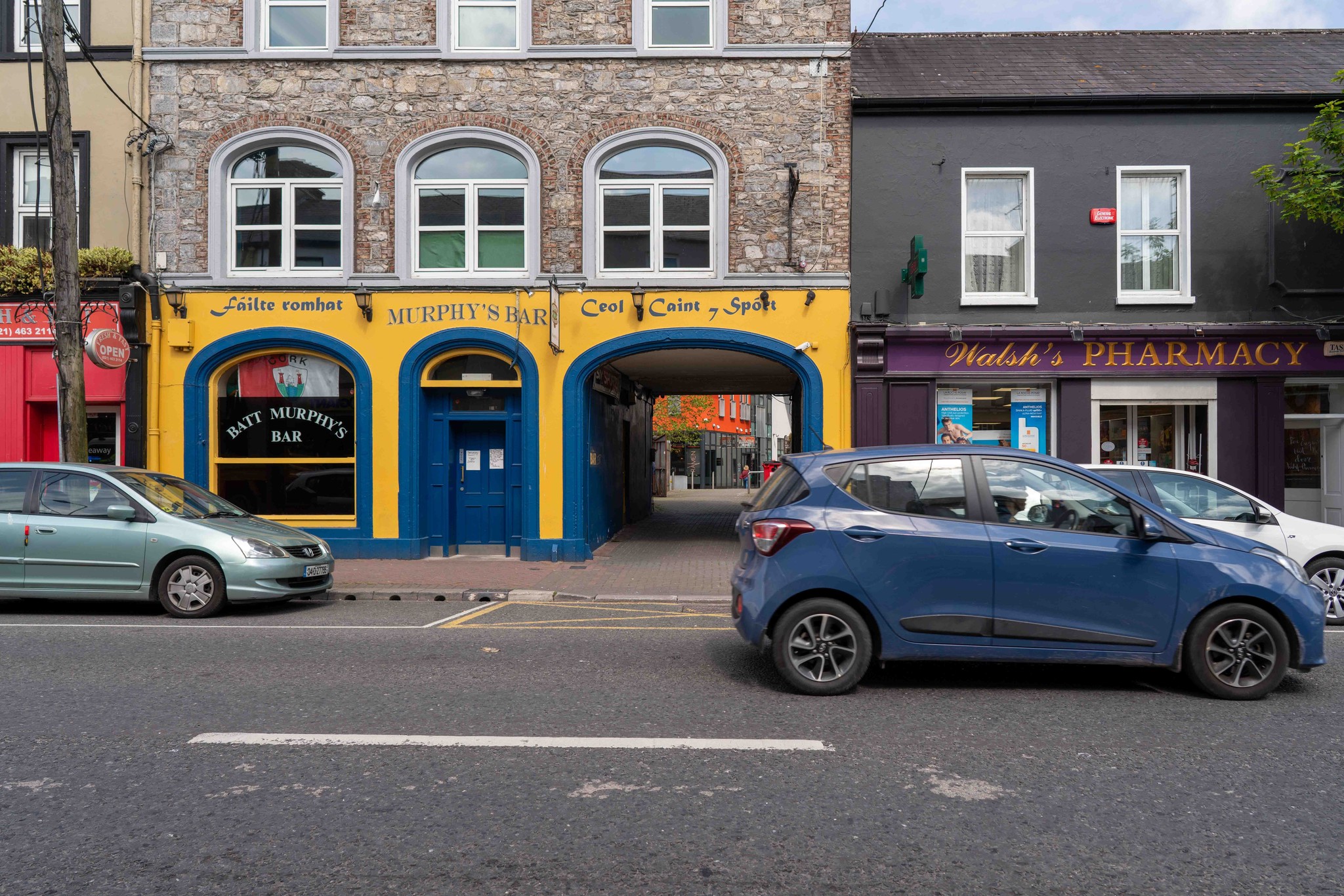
left=0, top=242, right=137, bottom=296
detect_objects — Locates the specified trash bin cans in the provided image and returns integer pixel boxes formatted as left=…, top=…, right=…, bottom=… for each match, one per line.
left=761, top=461, right=782, bottom=483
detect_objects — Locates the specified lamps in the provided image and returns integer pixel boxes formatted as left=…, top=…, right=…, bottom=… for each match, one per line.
left=736, top=427, right=743, bottom=432
left=948, top=325, right=962, bottom=341
left=773, top=432, right=779, bottom=437
left=713, top=424, right=720, bottom=429
left=805, top=291, right=815, bottom=306
left=352, top=283, right=372, bottom=322
left=1316, top=326, right=1330, bottom=340
left=164, top=281, right=187, bottom=319
left=760, top=291, right=769, bottom=307
left=1066, top=324, right=1084, bottom=341
left=1185, top=323, right=1204, bottom=337
left=557, top=282, right=586, bottom=295
left=523, top=286, right=550, bottom=298
left=630, top=282, right=646, bottom=321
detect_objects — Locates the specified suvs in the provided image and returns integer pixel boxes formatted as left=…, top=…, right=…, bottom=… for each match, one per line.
left=0, top=461, right=337, bottom=616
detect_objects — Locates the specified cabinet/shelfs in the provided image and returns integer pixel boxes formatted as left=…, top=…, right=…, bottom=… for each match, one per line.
left=1100, top=420, right=1126, bottom=464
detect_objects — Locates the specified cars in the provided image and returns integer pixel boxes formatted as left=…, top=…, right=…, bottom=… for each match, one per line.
left=1014, top=464, right=1344, bottom=627
left=729, top=444, right=1327, bottom=697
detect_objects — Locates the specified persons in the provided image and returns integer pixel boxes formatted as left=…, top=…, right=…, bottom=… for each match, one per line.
left=739, top=465, right=752, bottom=489
left=998, top=440, right=1011, bottom=447
left=937, top=417, right=972, bottom=445
left=997, top=473, right=1028, bottom=524
